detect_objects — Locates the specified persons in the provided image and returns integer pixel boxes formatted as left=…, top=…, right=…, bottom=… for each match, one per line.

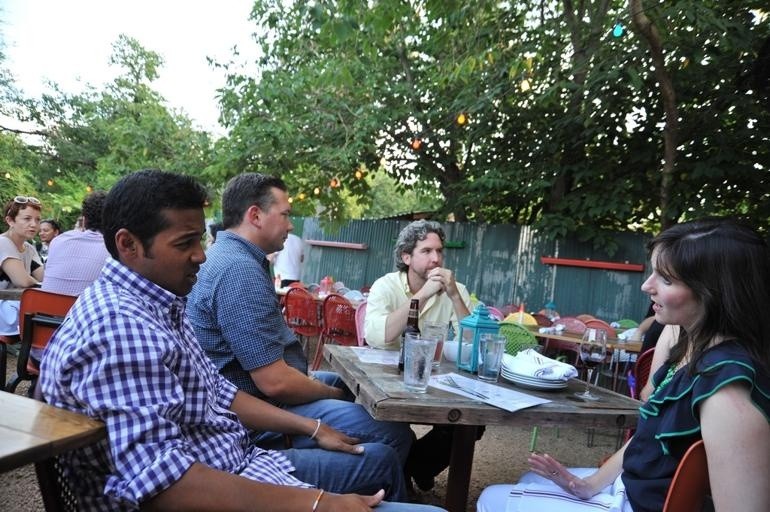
left=477, top=217, right=770, bottom=510
left=39, top=220, right=61, bottom=243
left=634, top=317, right=664, bottom=360
left=270, top=232, right=305, bottom=289
left=363, top=220, right=486, bottom=491
left=30, top=191, right=112, bottom=370
left=0, top=198, right=43, bottom=339
left=184, top=171, right=416, bottom=474
left=41, top=171, right=446, bottom=511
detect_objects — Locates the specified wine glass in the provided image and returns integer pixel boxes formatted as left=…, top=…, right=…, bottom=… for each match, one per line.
left=573, top=327, right=608, bottom=401
left=40, top=242, right=50, bottom=264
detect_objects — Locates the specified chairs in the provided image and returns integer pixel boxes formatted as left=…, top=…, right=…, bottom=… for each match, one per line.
left=8, top=288, right=75, bottom=396
left=278, top=280, right=656, bottom=451
left=661, top=440, right=713, bottom=510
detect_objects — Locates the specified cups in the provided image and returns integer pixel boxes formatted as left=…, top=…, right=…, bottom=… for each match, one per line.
left=423, top=319, right=450, bottom=370
left=537, top=324, right=567, bottom=335
left=403, top=333, right=437, bottom=395
left=478, top=332, right=507, bottom=383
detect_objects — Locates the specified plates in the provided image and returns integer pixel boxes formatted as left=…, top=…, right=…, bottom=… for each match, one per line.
left=499, top=354, right=569, bottom=392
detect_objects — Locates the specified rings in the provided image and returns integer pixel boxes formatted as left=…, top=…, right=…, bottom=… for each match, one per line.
left=552, top=472, right=559, bottom=477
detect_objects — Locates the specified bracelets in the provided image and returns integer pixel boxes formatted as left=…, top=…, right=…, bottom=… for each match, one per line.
left=309, top=419, right=321, bottom=439
left=311, top=488, right=325, bottom=511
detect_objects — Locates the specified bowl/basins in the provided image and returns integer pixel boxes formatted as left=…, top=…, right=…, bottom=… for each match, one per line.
left=441, top=338, right=473, bottom=363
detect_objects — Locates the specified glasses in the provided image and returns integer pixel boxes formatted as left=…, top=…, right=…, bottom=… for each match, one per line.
left=7, top=196, right=42, bottom=216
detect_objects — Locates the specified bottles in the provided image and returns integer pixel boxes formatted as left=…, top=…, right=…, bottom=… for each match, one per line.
left=274, top=274, right=282, bottom=290
left=397, top=298, right=421, bottom=372
left=319, top=276, right=331, bottom=297
left=544, top=301, right=555, bottom=321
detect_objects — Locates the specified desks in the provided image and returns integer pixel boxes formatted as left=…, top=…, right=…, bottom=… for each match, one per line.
left=0, top=287, right=33, bottom=390
left=2, top=395, right=107, bottom=512
